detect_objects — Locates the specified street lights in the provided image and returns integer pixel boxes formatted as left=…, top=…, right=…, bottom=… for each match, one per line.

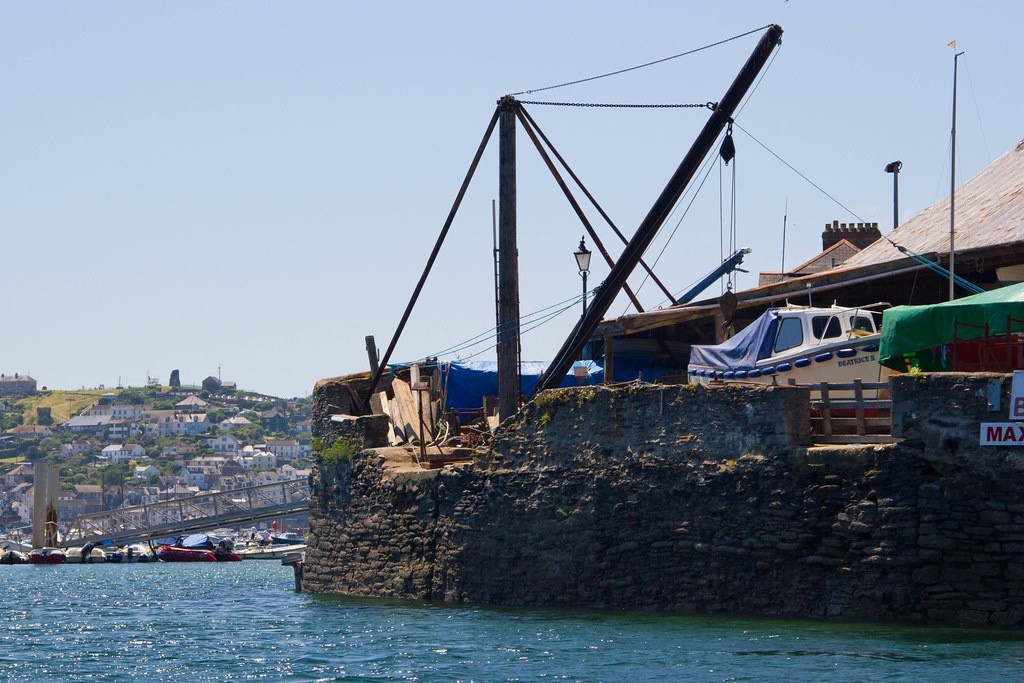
left=884, top=159, right=904, bottom=229
left=574, top=235, right=594, bottom=317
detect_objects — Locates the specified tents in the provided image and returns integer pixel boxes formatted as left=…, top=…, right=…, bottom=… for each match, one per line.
left=877, top=281, right=1024, bottom=372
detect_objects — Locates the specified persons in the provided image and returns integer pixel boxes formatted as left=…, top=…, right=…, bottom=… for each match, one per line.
left=249, top=520, right=302, bottom=546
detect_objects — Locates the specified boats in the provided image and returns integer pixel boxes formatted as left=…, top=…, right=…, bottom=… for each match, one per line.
left=685, top=298, right=907, bottom=436
left=0, top=527, right=307, bottom=566
